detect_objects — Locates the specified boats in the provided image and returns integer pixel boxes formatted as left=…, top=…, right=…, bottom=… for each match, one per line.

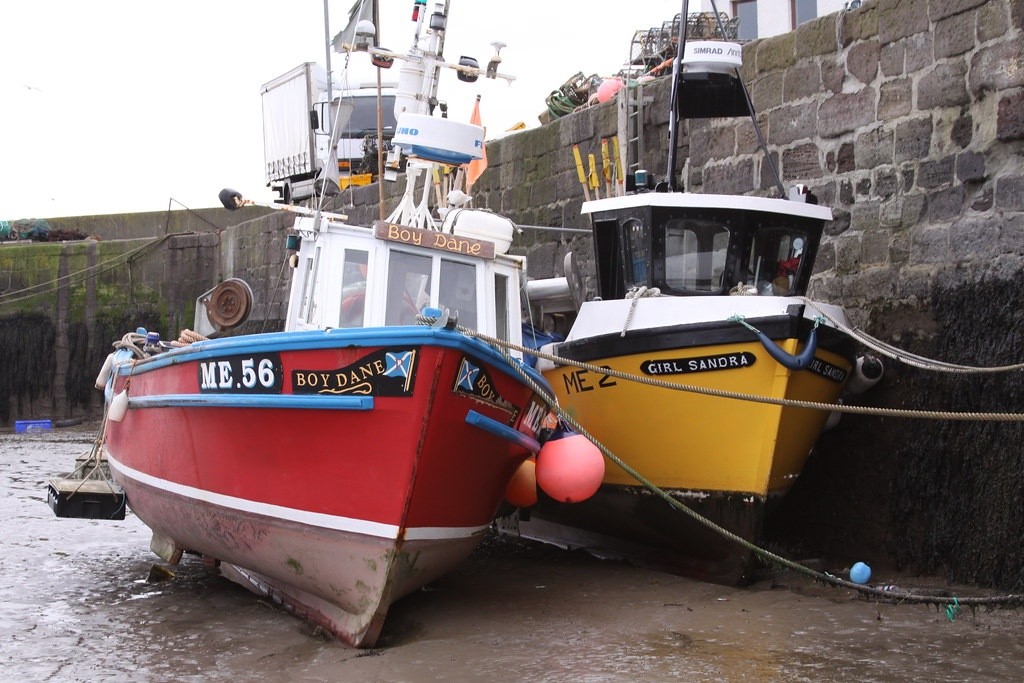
left=99, top=0, right=588, bottom=653
left=489, top=0, right=854, bottom=589
